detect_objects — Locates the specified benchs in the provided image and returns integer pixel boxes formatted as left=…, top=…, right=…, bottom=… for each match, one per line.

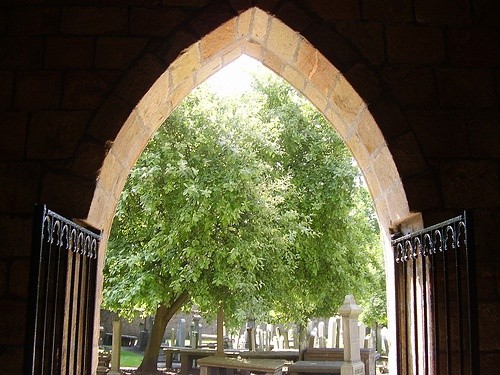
left=103, top=331, right=139, bottom=349
left=162, top=338, right=379, bottom=375
